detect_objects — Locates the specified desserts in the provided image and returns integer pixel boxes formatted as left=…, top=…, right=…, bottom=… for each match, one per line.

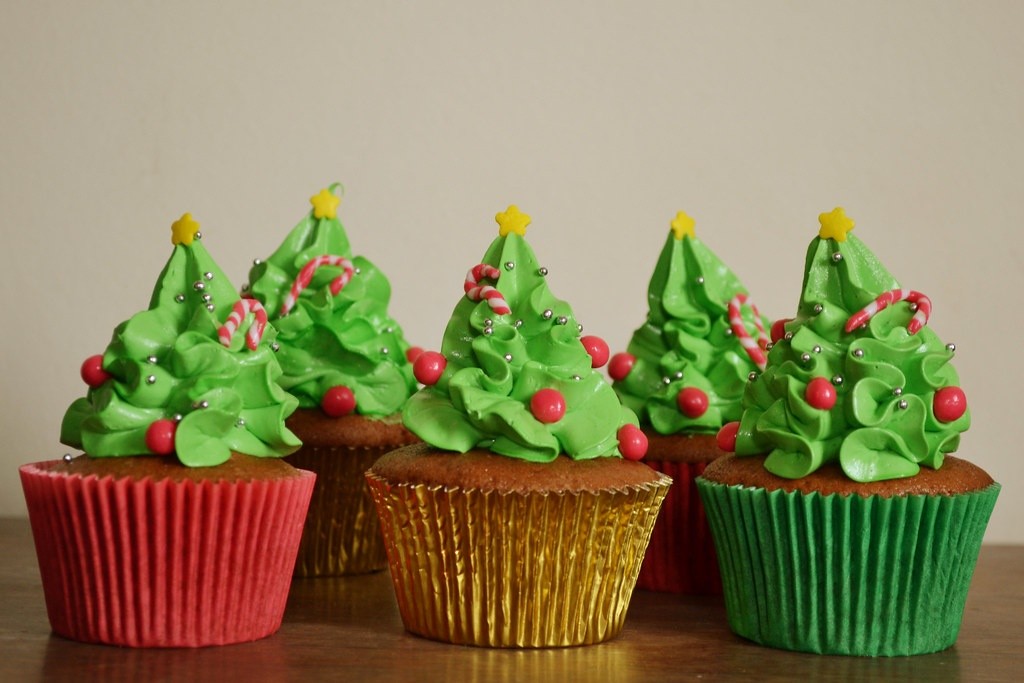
left=17, top=181, right=1001, bottom=658
